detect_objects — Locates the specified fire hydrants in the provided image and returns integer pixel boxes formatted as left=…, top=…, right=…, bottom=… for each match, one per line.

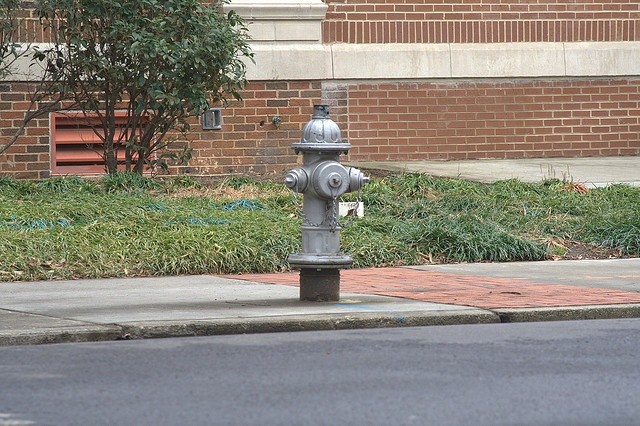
left=285, top=104, right=370, bottom=302
